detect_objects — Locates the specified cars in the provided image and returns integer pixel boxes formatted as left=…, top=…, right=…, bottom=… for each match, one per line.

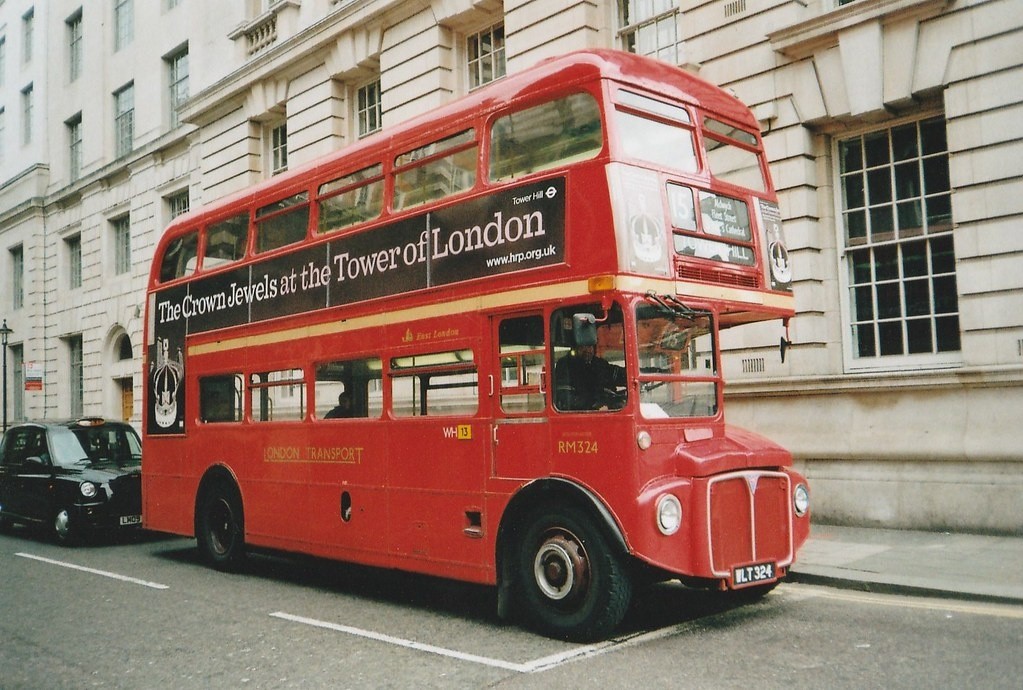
left=0, top=416, right=142, bottom=548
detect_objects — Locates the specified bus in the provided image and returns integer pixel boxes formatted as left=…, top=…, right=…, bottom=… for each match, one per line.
left=140, top=47, right=814, bottom=645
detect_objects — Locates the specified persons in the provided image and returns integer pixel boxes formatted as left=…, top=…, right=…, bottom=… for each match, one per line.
left=555, top=343, right=675, bottom=411
left=209, top=236, right=248, bottom=260
left=323, top=392, right=354, bottom=419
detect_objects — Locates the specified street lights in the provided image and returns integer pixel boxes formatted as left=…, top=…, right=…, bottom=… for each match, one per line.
left=0, top=318, right=15, bottom=433
